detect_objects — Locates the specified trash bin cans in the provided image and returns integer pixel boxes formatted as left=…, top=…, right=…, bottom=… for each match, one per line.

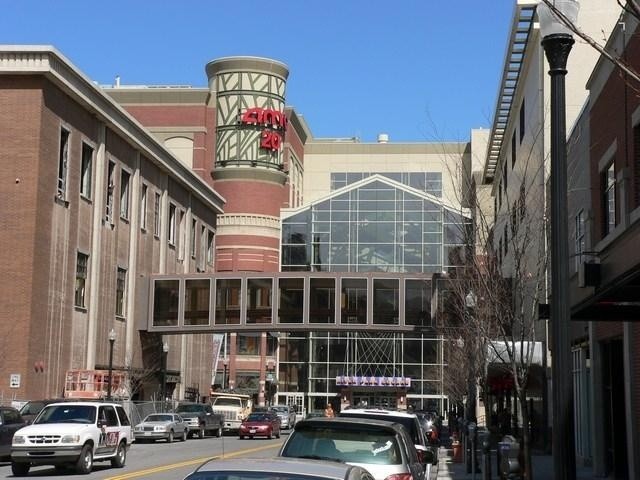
left=451, top=440, right=464, bottom=463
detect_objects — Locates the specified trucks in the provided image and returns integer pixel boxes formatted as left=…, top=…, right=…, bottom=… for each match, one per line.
left=211, top=395, right=252, bottom=435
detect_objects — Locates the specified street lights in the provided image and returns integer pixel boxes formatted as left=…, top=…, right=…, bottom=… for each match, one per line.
left=107, top=328, right=117, bottom=401
left=536, top=0, right=580, bottom=480
left=465, top=290, right=478, bottom=417
left=163, top=342, right=169, bottom=400
left=223, top=359, right=228, bottom=389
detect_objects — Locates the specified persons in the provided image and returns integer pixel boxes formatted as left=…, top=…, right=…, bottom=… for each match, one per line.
left=325, top=402, right=335, bottom=418
left=486, top=407, right=513, bottom=440
